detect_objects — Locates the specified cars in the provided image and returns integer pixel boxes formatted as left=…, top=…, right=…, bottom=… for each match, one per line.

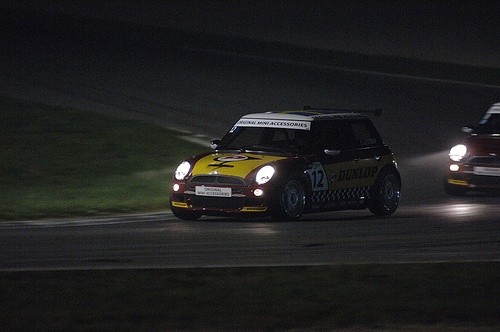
left=168, top=103, right=402, bottom=220
left=444, top=101, right=500, bottom=198
